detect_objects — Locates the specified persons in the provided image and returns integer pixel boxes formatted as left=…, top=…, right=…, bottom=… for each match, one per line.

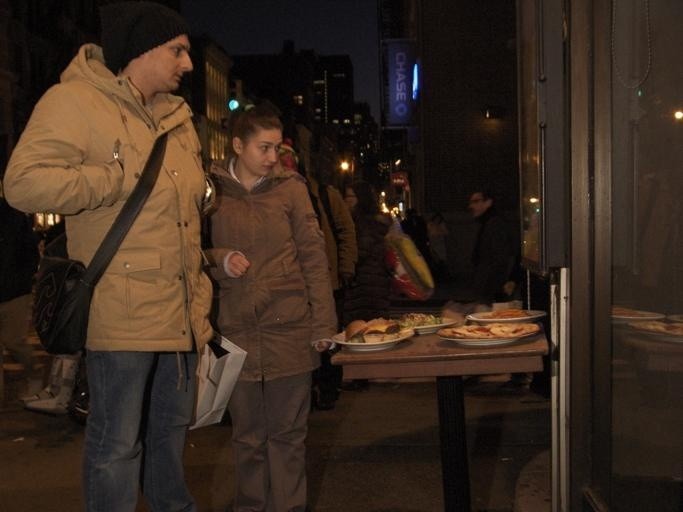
left=343, top=178, right=396, bottom=394
left=272, top=137, right=360, bottom=412
left=467, top=187, right=533, bottom=392
left=203, top=95, right=341, bottom=511
left=400, top=209, right=456, bottom=284
left=2, top=1, right=219, bottom=511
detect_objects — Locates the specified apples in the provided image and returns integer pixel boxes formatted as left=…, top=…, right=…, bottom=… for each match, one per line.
left=345, top=320, right=367, bottom=340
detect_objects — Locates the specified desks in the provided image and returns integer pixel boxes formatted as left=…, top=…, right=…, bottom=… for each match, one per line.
left=328, top=302, right=548, bottom=512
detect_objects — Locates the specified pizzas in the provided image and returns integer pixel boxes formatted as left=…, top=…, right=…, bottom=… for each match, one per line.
left=435, top=323, right=539, bottom=339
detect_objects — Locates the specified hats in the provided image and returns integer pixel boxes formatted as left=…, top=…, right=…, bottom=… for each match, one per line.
left=97, top=1, right=191, bottom=69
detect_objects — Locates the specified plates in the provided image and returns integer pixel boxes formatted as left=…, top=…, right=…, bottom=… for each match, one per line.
left=465, top=309, right=547, bottom=324
left=626, top=320, right=683, bottom=345
left=610, top=310, right=667, bottom=325
left=667, top=314, right=683, bottom=323
left=331, top=327, right=415, bottom=352
left=439, top=321, right=539, bottom=346
left=413, top=317, right=458, bottom=335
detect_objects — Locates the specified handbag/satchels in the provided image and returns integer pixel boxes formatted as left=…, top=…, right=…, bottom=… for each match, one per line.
left=187, top=325, right=250, bottom=431
left=30, top=249, right=97, bottom=355
